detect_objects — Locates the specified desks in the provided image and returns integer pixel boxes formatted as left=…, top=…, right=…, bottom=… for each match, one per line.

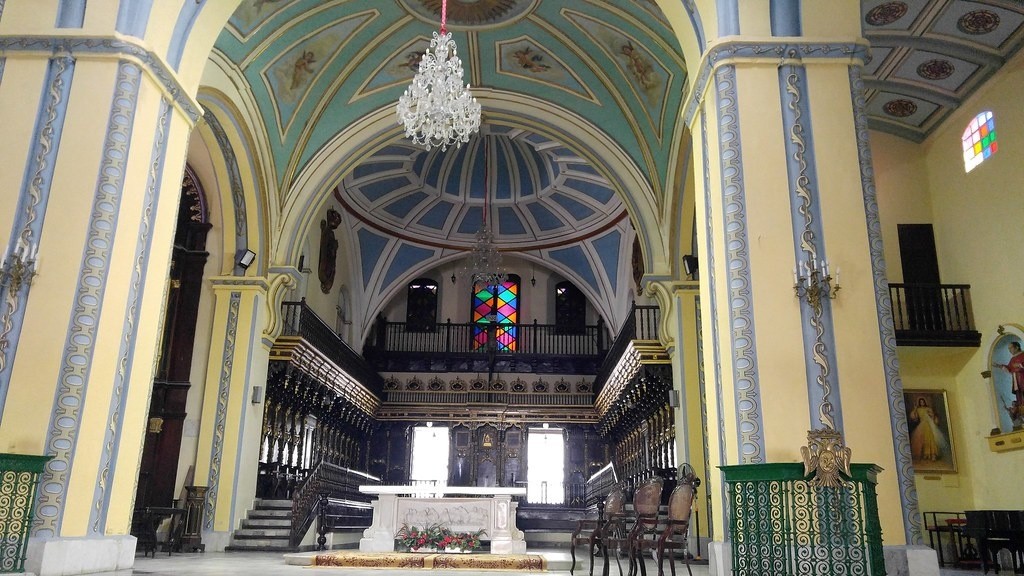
left=132, top=506, right=188, bottom=558
left=358, top=485, right=527, bottom=552
left=924, top=510, right=1024, bottom=574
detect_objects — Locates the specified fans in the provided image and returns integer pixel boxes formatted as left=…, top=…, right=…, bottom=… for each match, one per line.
left=676, top=464, right=708, bottom=564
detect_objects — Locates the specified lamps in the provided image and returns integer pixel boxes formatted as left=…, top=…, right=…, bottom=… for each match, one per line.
left=792, top=258, right=842, bottom=303
left=234, top=248, right=256, bottom=269
left=531, top=276, right=536, bottom=286
left=450, top=273, right=456, bottom=283
left=458, top=134, right=509, bottom=287
left=395, top=0, right=482, bottom=152
left=681, top=254, right=699, bottom=275
left=0, top=237, right=42, bottom=297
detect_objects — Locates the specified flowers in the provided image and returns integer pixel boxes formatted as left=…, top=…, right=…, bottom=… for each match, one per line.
left=395, top=522, right=489, bottom=552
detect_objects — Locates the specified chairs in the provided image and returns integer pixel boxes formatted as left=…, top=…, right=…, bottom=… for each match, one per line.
left=630, top=473, right=696, bottom=576
left=598, top=477, right=664, bottom=576
left=570, top=480, right=628, bottom=576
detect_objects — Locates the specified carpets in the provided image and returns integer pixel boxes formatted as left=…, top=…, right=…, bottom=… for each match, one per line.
left=303, top=549, right=550, bottom=573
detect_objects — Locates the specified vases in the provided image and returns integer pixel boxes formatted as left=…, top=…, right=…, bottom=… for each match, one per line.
left=410, top=546, right=472, bottom=554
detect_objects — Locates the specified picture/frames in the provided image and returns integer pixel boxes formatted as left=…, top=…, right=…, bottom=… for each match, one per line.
left=902, top=387, right=958, bottom=475
